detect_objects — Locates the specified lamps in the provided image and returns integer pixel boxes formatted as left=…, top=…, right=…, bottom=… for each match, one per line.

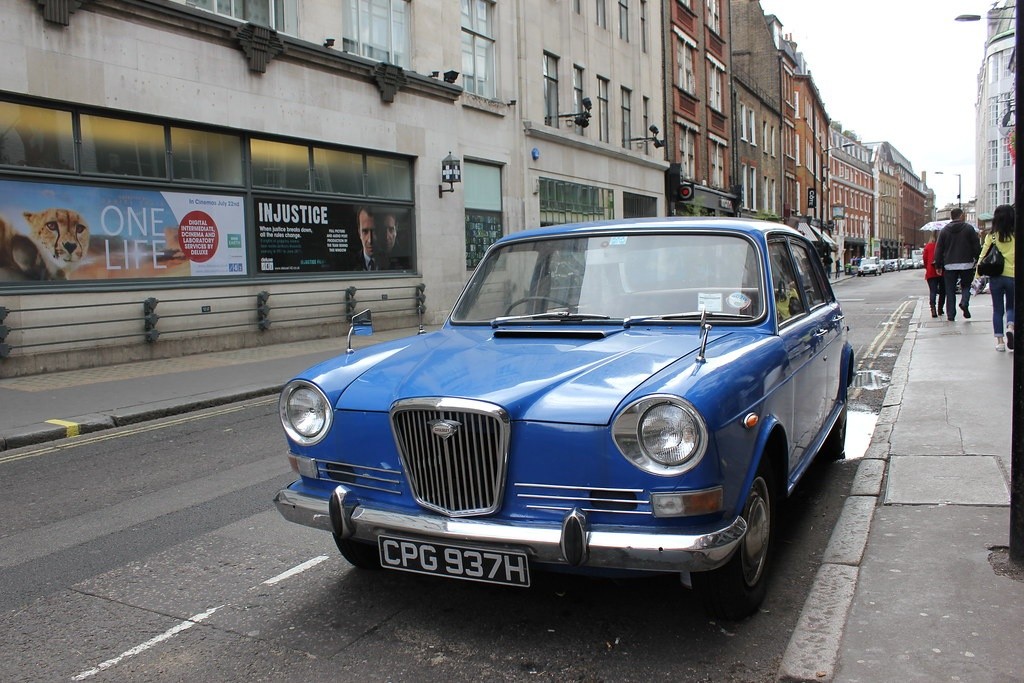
left=507, top=99, right=517, bottom=105
left=630, top=125, right=664, bottom=148
left=546, top=97, right=592, bottom=128
left=444, top=70, right=459, bottom=83
left=324, top=38, right=335, bottom=49
left=429, top=71, right=440, bottom=78
left=438, top=152, right=461, bottom=198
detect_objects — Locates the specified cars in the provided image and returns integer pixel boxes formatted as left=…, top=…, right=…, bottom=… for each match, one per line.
left=857, top=257, right=882, bottom=276
left=879, top=258, right=925, bottom=273
left=274, top=214, right=860, bottom=624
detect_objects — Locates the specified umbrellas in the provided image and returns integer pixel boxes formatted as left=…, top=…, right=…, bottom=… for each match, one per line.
left=919, top=219, right=980, bottom=233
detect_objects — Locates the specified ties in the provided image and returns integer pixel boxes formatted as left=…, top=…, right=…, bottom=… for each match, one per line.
left=369, top=260, right=376, bottom=270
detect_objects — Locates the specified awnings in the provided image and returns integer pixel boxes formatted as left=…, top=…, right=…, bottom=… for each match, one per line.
left=812, top=225, right=838, bottom=246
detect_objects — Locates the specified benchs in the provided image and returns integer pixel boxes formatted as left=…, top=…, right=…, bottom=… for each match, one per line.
left=628, top=288, right=792, bottom=324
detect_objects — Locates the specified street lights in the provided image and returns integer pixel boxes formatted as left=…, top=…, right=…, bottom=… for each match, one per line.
left=935, top=171, right=962, bottom=209
left=819, top=142, right=855, bottom=236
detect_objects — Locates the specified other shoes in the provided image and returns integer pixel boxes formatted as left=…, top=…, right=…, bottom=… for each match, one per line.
left=996, top=344, right=1005, bottom=351
left=948, top=318, right=954, bottom=321
left=960, top=304, right=970, bottom=318
left=1006, top=328, right=1014, bottom=350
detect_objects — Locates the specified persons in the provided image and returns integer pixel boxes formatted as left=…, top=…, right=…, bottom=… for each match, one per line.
left=933, top=208, right=982, bottom=320
left=922, top=229, right=946, bottom=317
left=825, top=255, right=834, bottom=277
left=350, top=204, right=408, bottom=271
left=974, top=204, right=1016, bottom=353
left=836, top=257, right=841, bottom=278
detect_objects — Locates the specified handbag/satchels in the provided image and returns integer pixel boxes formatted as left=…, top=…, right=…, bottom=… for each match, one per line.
left=977, top=232, right=1005, bottom=277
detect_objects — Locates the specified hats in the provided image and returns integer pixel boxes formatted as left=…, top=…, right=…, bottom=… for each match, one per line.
left=951, top=209, right=962, bottom=220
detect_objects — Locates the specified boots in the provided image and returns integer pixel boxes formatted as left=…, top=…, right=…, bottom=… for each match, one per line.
left=938, top=300, right=944, bottom=315
left=931, top=303, right=937, bottom=317
